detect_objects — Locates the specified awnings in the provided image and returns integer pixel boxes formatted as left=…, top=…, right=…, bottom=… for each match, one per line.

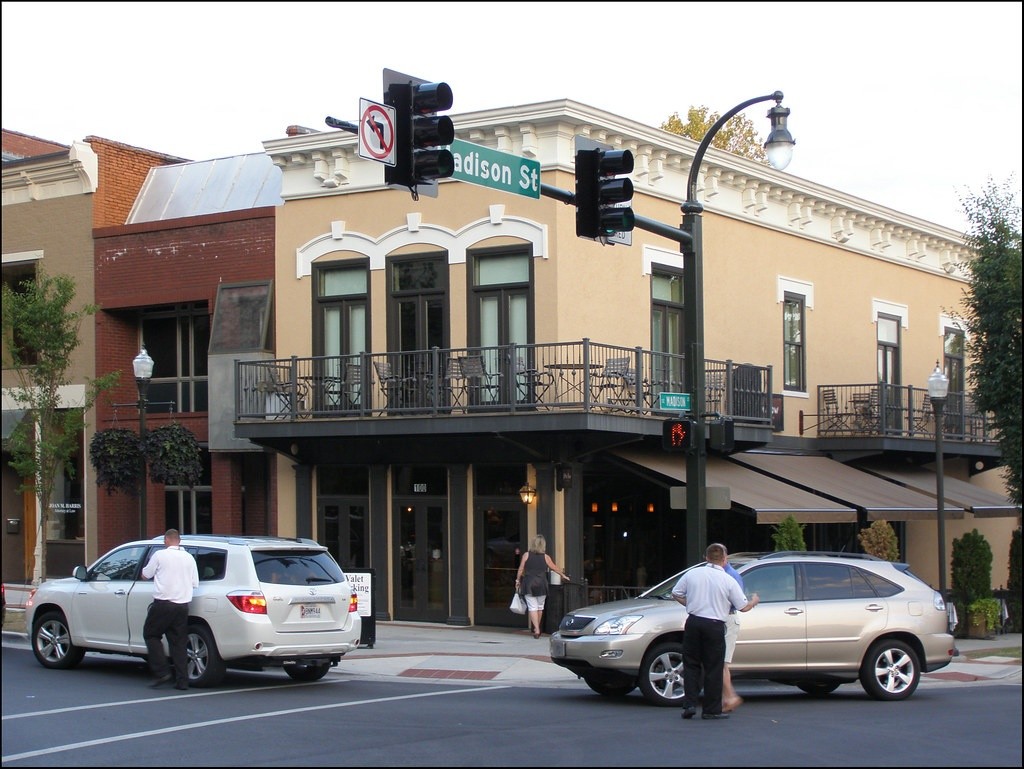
left=602, top=444, right=1023, bottom=524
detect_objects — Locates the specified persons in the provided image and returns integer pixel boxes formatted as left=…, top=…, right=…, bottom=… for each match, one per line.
left=140, top=529, right=199, bottom=690
left=721, top=544, right=744, bottom=714
left=671, top=544, right=761, bottom=719
left=515, top=535, right=570, bottom=639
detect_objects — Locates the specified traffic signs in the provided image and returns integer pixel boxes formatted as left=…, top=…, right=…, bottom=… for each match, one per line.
left=433, top=138, right=540, bottom=199
left=659, top=392, right=691, bottom=411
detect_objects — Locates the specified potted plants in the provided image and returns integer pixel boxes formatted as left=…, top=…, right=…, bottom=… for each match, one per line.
left=965, top=595, right=1003, bottom=639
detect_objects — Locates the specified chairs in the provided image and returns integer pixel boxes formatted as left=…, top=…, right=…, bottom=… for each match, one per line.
left=705, top=373, right=727, bottom=420
left=905, top=394, right=933, bottom=438
left=942, top=399, right=984, bottom=441
left=268, top=356, right=663, bottom=421
left=823, top=389, right=880, bottom=436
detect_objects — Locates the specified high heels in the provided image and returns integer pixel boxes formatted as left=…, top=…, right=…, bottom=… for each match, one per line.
left=534, top=632, right=541, bottom=639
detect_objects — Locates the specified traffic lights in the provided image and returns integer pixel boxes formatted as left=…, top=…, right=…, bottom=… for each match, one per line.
left=710, top=416, right=735, bottom=452
left=380, top=69, right=454, bottom=199
left=662, top=419, right=697, bottom=454
left=574, top=134, right=636, bottom=246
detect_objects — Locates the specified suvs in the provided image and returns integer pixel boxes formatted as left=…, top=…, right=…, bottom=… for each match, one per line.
left=24, top=534, right=363, bottom=687
left=551, top=551, right=960, bottom=708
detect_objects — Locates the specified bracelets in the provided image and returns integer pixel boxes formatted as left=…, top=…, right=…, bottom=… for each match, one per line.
left=515, top=580, right=519, bottom=582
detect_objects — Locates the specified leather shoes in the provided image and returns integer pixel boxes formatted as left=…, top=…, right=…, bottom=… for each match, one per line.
left=681, top=706, right=696, bottom=719
left=701, top=711, right=728, bottom=719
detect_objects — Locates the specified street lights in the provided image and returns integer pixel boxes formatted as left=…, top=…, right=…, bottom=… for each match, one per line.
left=681, top=91, right=797, bottom=566
left=927, top=359, right=960, bottom=657
left=132, top=343, right=155, bottom=541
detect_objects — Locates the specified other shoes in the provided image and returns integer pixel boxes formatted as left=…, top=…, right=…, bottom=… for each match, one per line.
left=145, top=674, right=171, bottom=688
left=720, top=696, right=743, bottom=713
left=174, top=682, right=189, bottom=690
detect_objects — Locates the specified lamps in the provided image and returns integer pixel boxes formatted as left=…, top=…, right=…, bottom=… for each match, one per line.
left=519, top=481, right=538, bottom=504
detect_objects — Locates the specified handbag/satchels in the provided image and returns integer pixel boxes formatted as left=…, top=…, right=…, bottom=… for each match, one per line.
left=509, top=586, right=526, bottom=615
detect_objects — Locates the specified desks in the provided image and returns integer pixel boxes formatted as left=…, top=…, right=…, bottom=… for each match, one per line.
left=544, top=363, right=603, bottom=409
left=296, top=375, right=345, bottom=417
